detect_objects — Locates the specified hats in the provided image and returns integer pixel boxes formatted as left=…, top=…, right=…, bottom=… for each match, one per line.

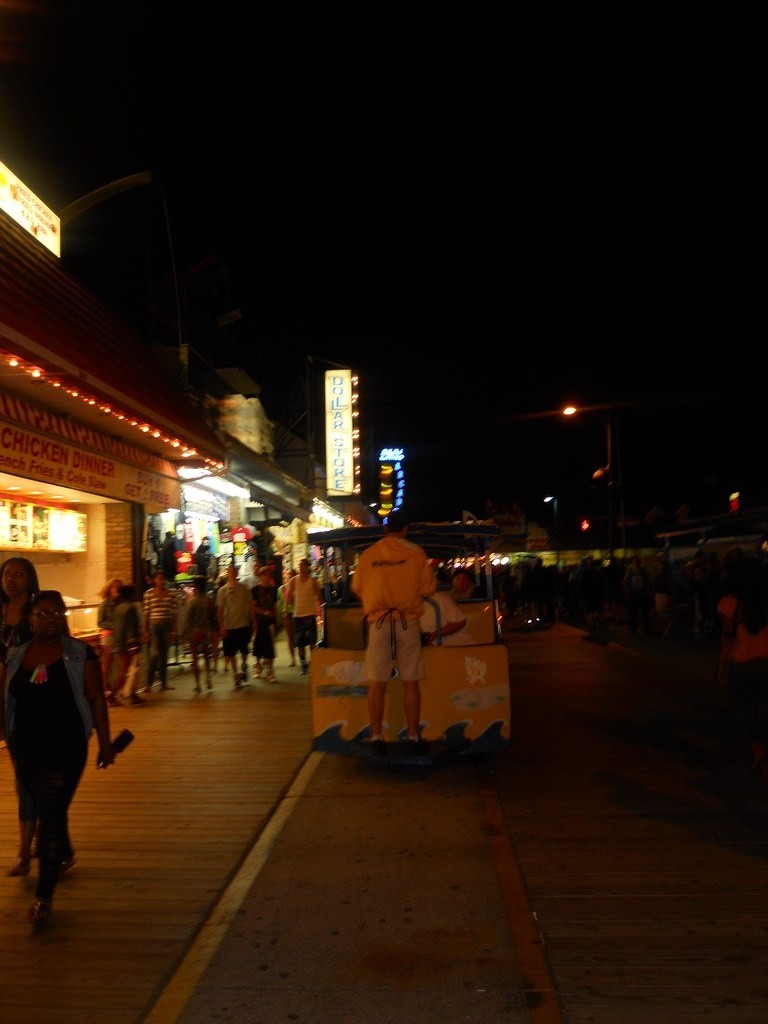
left=258, top=566, right=273, bottom=576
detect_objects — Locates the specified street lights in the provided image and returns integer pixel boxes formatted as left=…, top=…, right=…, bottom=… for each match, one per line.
left=562, top=406, right=613, bottom=562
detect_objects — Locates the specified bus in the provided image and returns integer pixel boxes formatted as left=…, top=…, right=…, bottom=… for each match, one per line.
left=305, top=518, right=515, bottom=752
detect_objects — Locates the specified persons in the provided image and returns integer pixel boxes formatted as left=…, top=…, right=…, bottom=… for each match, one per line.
left=451, top=569, right=473, bottom=599
left=0, top=592, right=113, bottom=927
left=0, top=558, right=69, bottom=876
left=98, top=560, right=324, bottom=706
left=350, top=511, right=437, bottom=742
left=492, top=550, right=768, bottom=784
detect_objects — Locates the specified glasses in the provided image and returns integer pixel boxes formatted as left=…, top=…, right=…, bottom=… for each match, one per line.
left=31, top=611, right=64, bottom=619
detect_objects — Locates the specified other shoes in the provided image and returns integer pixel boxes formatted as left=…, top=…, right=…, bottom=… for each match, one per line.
left=107, top=653, right=308, bottom=706
left=407, top=739, right=430, bottom=755
left=32, top=832, right=39, bottom=858
left=362, top=738, right=385, bottom=754
left=56, top=856, right=78, bottom=877
left=8, top=857, right=29, bottom=875
left=29, top=901, right=52, bottom=924
